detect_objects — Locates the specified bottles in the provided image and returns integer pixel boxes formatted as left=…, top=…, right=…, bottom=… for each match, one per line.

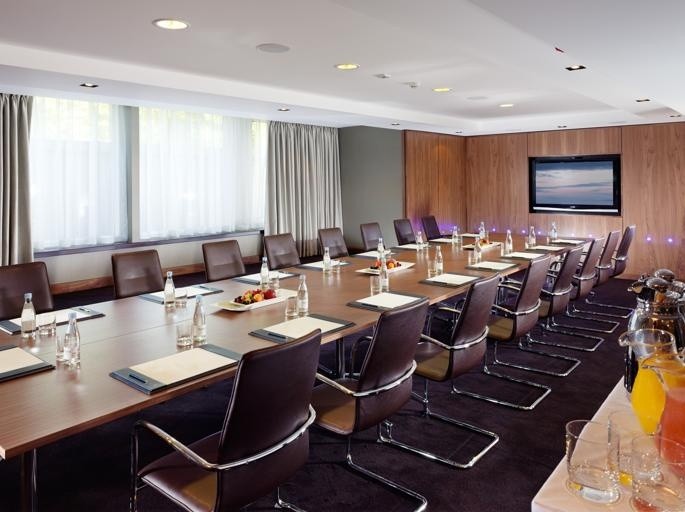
left=474, top=236, right=481, bottom=262
left=163, top=271, right=175, bottom=308
left=191, top=294, right=208, bottom=347
left=479, top=222, right=485, bottom=240
left=379, top=258, right=390, bottom=292
left=297, top=275, right=309, bottom=313
left=260, top=257, right=270, bottom=285
left=505, top=221, right=557, bottom=253
left=63, top=312, right=81, bottom=367
left=20, top=292, right=36, bottom=338
left=452, top=226, right=458, bottom=245
left=377, top=236, right=386, bottom=256
left=323, top=245, right=331, bottom=273
left=435, top=245, right=443, bottom=273
left=415, top=231, right=424, bottom=251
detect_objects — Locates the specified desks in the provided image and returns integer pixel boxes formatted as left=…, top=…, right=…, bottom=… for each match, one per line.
left=529, top=371, right=684, bottom=512
left=0, top=229, right=600, bottom=511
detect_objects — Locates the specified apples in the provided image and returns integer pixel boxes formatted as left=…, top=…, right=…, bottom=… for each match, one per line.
left=264, top=289, right=275, bottom=299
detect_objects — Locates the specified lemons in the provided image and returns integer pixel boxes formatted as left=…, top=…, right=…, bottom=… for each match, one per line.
left=253, top=293, right=263, bottom=302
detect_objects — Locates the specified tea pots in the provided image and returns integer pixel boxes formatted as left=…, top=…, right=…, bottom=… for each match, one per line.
left=622, top=264, right=685, bottom=388
left=640, top=351, right=684, bottom=480
left=617, top=329, right=682, bottom=432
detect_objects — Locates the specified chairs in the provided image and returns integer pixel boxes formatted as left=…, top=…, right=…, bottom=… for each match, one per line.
left=201, top=239, right=246, bottom=282
left=1, top=261, right=53, bottom=319
left=109, top=248, right=166, bottom=299
left=261, top=232, right=303, bottom=269
left=420, top=217, right=442, bottom=244
left=450, top=221, right=638, bottom=411
left=376, top=269, right=507, bottom=469
left=392, top=217, right=418, bottom=249
left=317, top=227, right=349, bottom=259
left=312, top=295, right=431, bottom=512
left=128, top=328, right=321, bottom=512
left=359, top=221, right=387, bottom=251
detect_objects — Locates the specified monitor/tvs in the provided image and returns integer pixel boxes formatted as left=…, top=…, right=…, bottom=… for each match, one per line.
left=528, top=153, right=621, bottom=217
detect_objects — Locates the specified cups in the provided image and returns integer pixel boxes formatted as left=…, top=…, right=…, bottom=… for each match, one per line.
left=284, top=298, right=299, bottom=317
left=55, top=336, right=65, bottom=362
left=427, top=259, right=438, bottom=277
left=270, top=274, right=280, bottom=288
left=458, top=235, right=464, bottom=244
left=486, top=231, right=490, bottom=240
left=177, top=287, right=188, bottom=308
left=385, top=248, right=392, bottom=257
left=423, top=242, right=430, bottom=249
left=37, top=314, right=57, bottom=335
left=562, top=418, right=621, bottom=504
left=605, top=408, right=663, bottom=486
left=468, top=251, right=477, bottom=265
left=369, top=275, right=381, bottom=292
left=174, top=323, right=193, bottom=346
left=626, top=433, right=685, bottom=511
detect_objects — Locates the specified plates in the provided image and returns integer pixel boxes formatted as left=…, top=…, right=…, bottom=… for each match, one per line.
left=355, top=261, right=416, bottom=276
left=461, top=241, right=501, bottom=248
left=213, top=286, right=300, bottom=312
